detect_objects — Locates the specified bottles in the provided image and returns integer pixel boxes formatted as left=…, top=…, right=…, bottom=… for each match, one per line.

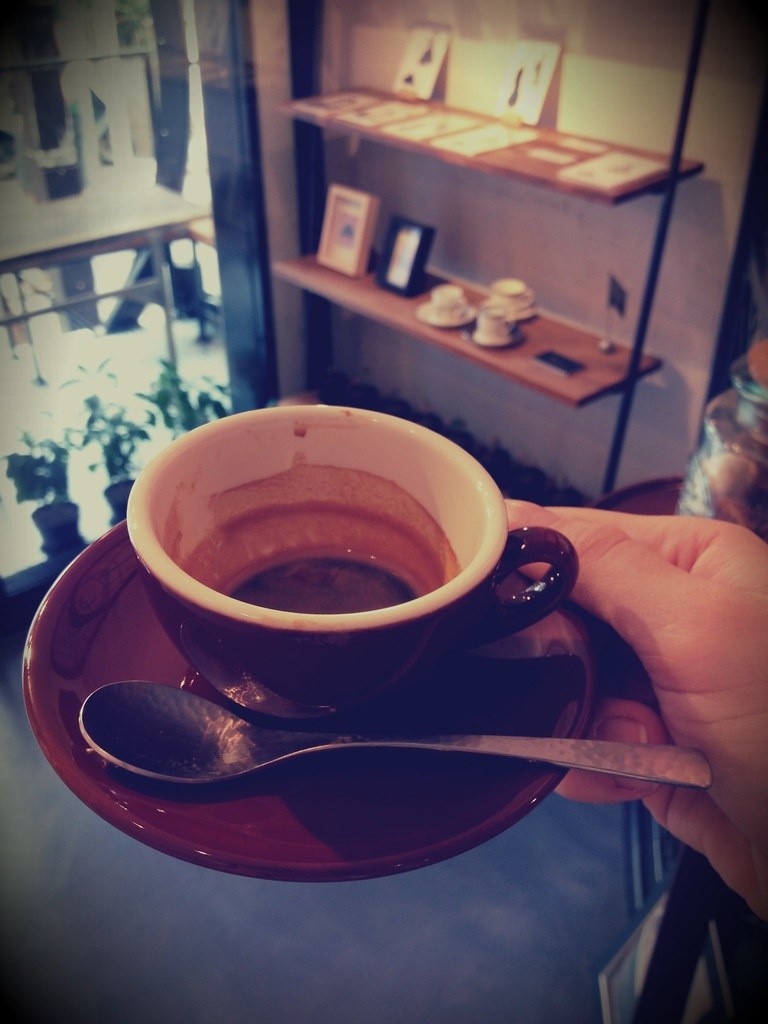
left=673, top=338, right=768, bottom=542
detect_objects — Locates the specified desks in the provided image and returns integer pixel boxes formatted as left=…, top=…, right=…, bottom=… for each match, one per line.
left=1, top=176, right=211, bottom=386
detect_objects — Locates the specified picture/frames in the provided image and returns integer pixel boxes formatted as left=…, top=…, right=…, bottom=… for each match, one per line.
left=315, top=183, right=383, bottom=277
left=375, top=213, right=437, bottom=298
left=492, top=39, right=562, bottom=126
left=391, top=25, right=452, bottom=100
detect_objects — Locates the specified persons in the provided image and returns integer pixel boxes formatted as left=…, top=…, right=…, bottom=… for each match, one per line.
left=503, top=500, right=767, bottom=921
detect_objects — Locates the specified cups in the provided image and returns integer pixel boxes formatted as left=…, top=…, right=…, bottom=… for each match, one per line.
left=477, top=300, right=517, bottom=337
left=431, top=284, right=467, bottom=320
left=127, top=404, right=579, bottom=717
left=491, top=279, right=535, bottom=311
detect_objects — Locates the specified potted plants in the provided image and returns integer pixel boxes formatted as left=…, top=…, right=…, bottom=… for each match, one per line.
left=5, top=355, right=230, bottom=563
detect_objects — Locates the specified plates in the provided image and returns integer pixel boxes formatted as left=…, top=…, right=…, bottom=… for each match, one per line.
left=417, top=303, right=477, bottom=327
left=478, top=299, right=534, bottom=321
left=466, top=321, right=525, bottom=349
left=22, top=520, right=594, bottom=882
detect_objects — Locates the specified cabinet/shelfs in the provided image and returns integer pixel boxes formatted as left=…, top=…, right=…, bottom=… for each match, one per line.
left=266, top=0, right=708, bottom=507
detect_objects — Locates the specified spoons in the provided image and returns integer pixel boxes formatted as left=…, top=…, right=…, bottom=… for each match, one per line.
left=81, top=681, right=712, bottom=787
